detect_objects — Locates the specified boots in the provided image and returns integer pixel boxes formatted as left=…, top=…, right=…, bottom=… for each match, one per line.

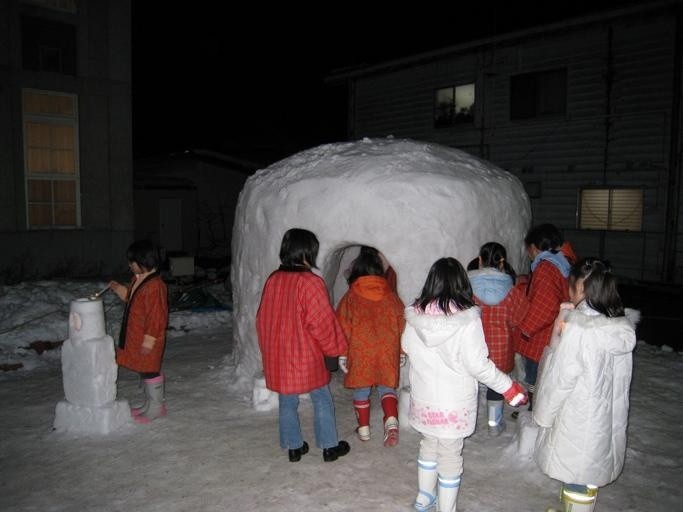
left=352, top=397, right=373, bottom=442
left=486, top=398, right=507, bottom=438
left=545, top=485, right=598, bottom=512
left=380, top=391, right=400, bottom=448
left=134, top=372, right=168, bottom=424
left=414, top=458, right=438, bottom=512
left=130, top=383, right=150, bottom=417
left=437, top=474, right=461, bottom=512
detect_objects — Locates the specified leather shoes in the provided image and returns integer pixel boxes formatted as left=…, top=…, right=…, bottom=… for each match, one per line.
left=288, top=440, right=310, bottom=463
left=322, top=439, right=351, bottom=462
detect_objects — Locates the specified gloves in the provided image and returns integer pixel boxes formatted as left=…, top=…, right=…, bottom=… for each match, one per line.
left=399, top=353, right=407, bottom=367
left=338, top=356, right=350, bottom=374
left=502, top=381, right=530, bottom=409
left=324, top=355, right=340, bottom=373
left=520, top=331, right=531, bottom=342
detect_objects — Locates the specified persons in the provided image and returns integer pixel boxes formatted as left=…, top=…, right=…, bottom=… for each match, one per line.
left=253, top=228, right=348, bottom=462
left=110, top=241, right=167, bottom=423
left=336, top=246, right=406, bottom=447
left=512, top=227, right=576, bottom=419
left=532, top=255, right=640, bottom=512
left=397, top=256, right=529, bottom=511
left=467, top=242, right=527, bottom=436
